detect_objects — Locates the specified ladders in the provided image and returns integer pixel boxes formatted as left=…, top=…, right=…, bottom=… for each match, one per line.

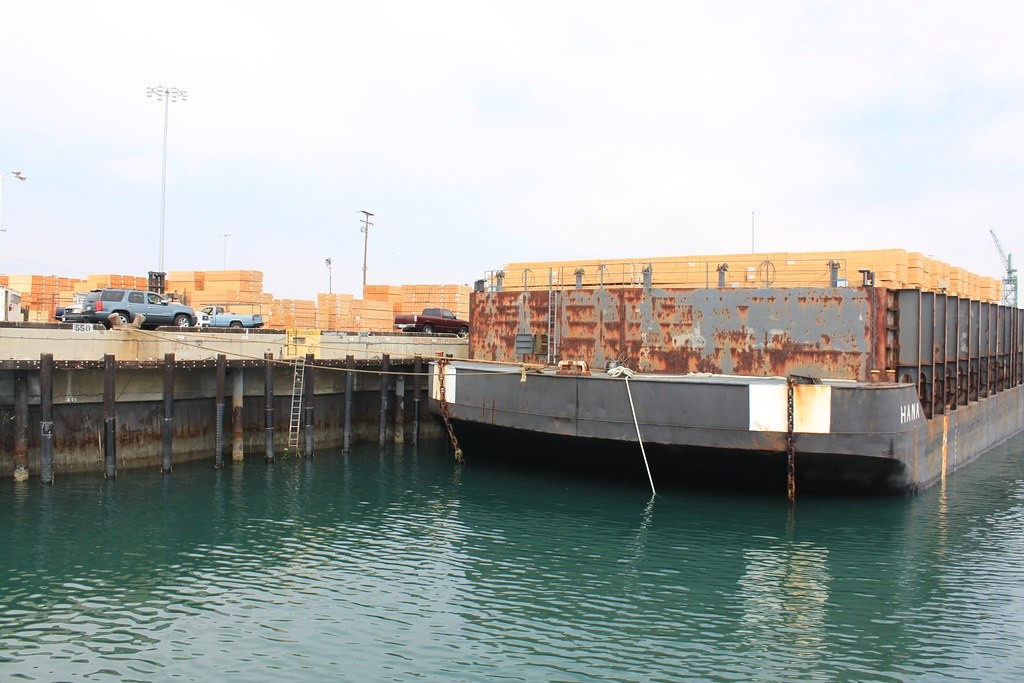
left=289, top=358, right=306, bottom=448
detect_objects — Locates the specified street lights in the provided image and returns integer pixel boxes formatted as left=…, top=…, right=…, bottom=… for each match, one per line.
left=220, top=233, right=231, bottom=270
left=751, top=212, right=754, bottom=253
left=146, top=84, right=187, bottom=271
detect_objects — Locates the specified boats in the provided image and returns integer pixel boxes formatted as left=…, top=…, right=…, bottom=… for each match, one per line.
left=428, top=257, right=1024, bottom=498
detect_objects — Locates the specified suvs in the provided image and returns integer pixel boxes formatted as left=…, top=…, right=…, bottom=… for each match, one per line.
left=64, top=286, right=211, bottom=330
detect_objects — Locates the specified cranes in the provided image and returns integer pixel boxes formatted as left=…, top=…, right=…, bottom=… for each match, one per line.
left=990, top=230, right=1018, bottom=307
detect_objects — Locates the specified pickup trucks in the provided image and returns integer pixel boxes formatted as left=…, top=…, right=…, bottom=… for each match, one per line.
left=201, top=306, right=264, bottom=329
left=392, top=306, right=469, bottom=336
